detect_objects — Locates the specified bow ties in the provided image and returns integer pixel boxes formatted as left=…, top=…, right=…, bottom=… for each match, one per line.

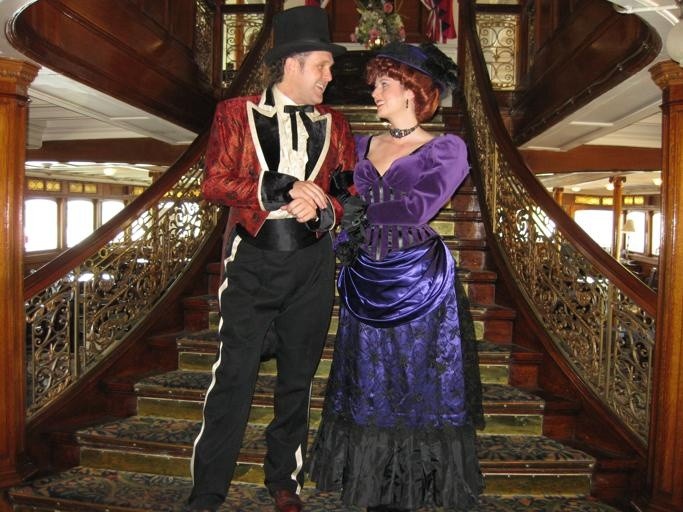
left=282, top=105, right=314, bottom=150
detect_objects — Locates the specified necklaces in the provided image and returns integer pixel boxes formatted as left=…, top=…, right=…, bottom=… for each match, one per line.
left=384, top=121, right=420, bottom=139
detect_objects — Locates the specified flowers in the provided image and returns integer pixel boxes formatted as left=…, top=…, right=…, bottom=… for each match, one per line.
left=349, top=0, right=405, bottom=49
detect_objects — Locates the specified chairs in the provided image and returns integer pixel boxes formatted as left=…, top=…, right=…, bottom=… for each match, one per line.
left=623, top=267, right=658, bottom=328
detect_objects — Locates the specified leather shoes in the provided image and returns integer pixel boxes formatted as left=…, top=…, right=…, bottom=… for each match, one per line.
left=275, top=489, right=302, bottom=512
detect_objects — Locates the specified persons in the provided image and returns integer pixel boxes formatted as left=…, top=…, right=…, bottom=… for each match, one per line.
left=187, top=1, right=359, bottom=512
left=300, top=36, right=487, bottom=512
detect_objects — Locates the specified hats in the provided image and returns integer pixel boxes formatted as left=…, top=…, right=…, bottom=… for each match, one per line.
left=374, top=42, right=448, bottom=99
left=265, top=6, right=347, bottom=66
